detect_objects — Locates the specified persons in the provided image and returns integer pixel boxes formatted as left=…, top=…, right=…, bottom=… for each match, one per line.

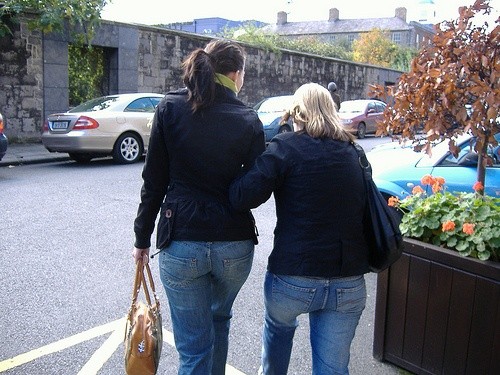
left=229, top=82, right=372, bottom=375
left=328, top=82, right=340, bottom=112
left=131, top=37, right=267, bottom=375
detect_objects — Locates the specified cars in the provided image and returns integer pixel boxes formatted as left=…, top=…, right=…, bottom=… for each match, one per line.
left=373, top=128, right=500, bottom=215
left=40, top=93, right=165, bottom=164
left=337, top=100, right=390, bottom=139
left=251, top=95, right=295, bottom=143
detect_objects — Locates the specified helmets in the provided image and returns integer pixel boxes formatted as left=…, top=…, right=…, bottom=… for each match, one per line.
left=328, top=82, right=337, bottom=90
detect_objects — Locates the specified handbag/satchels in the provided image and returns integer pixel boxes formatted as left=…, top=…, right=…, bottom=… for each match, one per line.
left=124, top=255, right=162, bottom=375
left=350, top=141, right=404, bottom=274
